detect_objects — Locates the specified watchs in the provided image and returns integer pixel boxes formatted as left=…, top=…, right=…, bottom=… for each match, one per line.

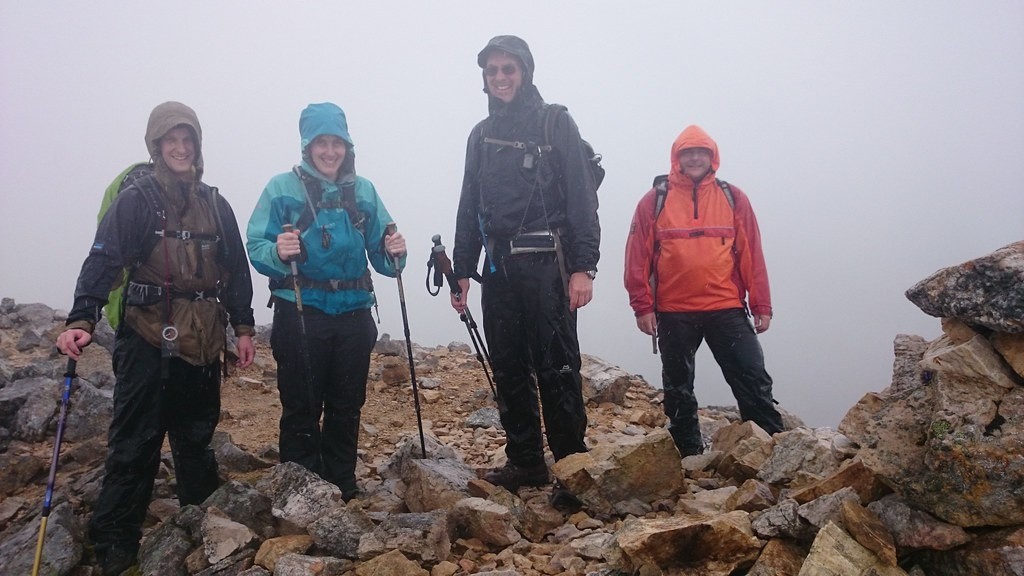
left=583, top=270, right=597, bottom=280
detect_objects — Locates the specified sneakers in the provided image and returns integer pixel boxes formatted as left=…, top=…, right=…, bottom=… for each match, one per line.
left=483, top=461, right=548, bottom=489
left=102, top=548, right=137, bottom=575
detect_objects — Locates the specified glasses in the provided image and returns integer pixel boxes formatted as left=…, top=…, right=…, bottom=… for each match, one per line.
left=485, top=63, right=515, bottom=77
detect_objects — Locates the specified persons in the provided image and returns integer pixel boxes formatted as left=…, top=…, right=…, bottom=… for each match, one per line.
left=623, top=125, right=791, bottom=455
left=246, top=103, right=413, bottom=498
left=56, top=100, right=256, bottom=573
left=448, top=33, right=606, bottom=491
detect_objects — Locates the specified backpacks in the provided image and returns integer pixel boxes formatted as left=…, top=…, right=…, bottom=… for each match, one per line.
left=479, top=107, right=605, bottom=190
left=98, top=162, right=157, bottom=329
